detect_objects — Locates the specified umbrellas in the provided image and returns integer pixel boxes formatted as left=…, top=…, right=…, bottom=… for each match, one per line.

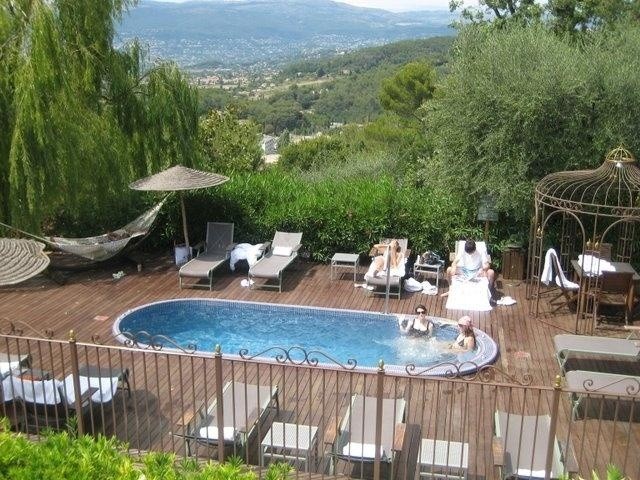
left=128, top=163, right=230, bottom=261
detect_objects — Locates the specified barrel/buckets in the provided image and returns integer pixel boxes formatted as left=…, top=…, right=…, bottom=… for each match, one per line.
left=174, top=243, right=190, bottom=266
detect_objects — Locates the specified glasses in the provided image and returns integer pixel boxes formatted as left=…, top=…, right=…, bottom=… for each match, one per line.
left=417, top=312, right=426, bottom=315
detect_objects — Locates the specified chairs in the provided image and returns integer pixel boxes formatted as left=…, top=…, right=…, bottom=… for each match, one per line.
left=553, top=331, right=640, bottom=371
left=541, top=247, right=588, bottom=314
left=0, top=353, right=132, bottom=440
left=171, top=378, right=279, bottom=467
left=563, top=369, right=640, bottom=420
left=322, top=394, right=413, bottom=480
left=446, top=240, right=493, bottom=312
left=178, top=221, right=304, bottom=293
left=583, top=242, right=613, bottom=262
left=589, top=270, right=634, bottom=328
left=363, top=238, right=411, bottom=300
left=491, top=410, right=579, bottom=480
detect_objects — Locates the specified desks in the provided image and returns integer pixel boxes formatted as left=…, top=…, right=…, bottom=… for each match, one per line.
left=261, top=422, right=319, bottom=473
left=331, top=253, right=360, bottom=282
left=414, top=439, right=469, bottom=480
left=414, top=259, right=445, bottom=288
left=569, top=260, right=640, bottom=318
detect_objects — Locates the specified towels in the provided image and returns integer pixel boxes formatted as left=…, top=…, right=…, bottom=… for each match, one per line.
left=342, top=442, right=383, bottom=460
left=516, top=469, right=553, bottom=480
left=403, top=277, right=438, bottom=296
left=199, top=426, right=236, bottom=440
left=541, top=248, right=580, bottom=290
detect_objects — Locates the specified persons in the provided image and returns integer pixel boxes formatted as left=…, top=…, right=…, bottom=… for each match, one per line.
left=49, top=230, right=131, bottom=245
left=452, top=316, right=477, bottom=354
left=440, top=238, right=495, bottom=299
left=372, top=240, right=401, bottom=277
left=395, top=305, right=434, bottom=339
